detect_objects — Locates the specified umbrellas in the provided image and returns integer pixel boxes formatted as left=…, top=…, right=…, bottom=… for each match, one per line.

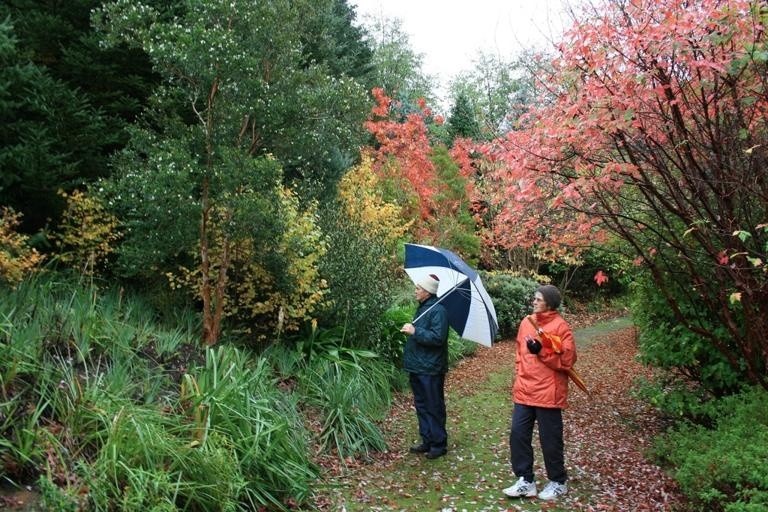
left=403, top=242, right=499, bottom=348
left=527, top=315, right=589, bottom=395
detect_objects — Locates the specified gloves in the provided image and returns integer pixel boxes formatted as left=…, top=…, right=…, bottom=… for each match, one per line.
left=526, top=338, right=542, bottom=355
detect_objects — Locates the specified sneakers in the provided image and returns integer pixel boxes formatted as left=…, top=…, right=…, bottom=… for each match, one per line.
left=425, top=448, right=447, bottom=458
left=409, top=442, right=431, bottom=452
left=537, top=480, right=568, bottom=500
left=502, top=476, right=537, bottom=497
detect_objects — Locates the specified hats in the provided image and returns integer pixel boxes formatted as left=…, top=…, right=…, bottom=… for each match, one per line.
left=536, top=285, right=562, bottom=311
left=417, top=274, right=440, bottom=295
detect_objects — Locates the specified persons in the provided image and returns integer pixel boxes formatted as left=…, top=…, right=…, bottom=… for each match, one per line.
left=502, top=284, right=577, bottom=501
left=399, top=274, right=449, bottom=459
